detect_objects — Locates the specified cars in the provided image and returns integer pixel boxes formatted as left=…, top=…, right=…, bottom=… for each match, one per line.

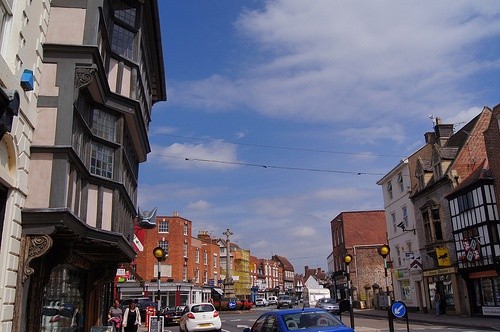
left=158, top=306, right=184, bottom=326
left=179, top=303, right=222, bottom=332
left=315, top=297, right=339, bottom=315
left=41, top=306, right=78, bottom=332
left=242, top=307, right=354, bottom=332
left=255, top=295, right=299, bottom=308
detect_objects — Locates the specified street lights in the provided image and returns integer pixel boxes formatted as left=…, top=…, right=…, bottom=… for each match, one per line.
left=343, top=254, right=355, bottom=329
left=377, top=244, right=395, bottom=332
left=152, top=246, right=166, bottom=321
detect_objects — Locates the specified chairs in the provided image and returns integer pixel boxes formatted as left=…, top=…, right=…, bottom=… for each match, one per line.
left=301, top=315, right=311, bottom=327
left=287, top=317, right=293, bottom=329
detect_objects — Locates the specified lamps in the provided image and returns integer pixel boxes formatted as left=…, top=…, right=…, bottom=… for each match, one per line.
left=397, top=222, right=415, bottom=234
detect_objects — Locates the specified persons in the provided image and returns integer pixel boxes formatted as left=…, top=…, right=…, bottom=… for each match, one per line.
left=432, top=290, right=443, bottom=315
left=122, top=302, right=141, bottom=332
left=108, top=300, right=123, bottom=332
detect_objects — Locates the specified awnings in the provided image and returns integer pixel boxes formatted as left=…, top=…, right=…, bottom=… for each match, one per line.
left=213, top=288, right=224, bottom=295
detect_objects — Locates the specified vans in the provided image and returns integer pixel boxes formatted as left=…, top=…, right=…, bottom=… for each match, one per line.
left=118, top=296, right=158, bottom=322
